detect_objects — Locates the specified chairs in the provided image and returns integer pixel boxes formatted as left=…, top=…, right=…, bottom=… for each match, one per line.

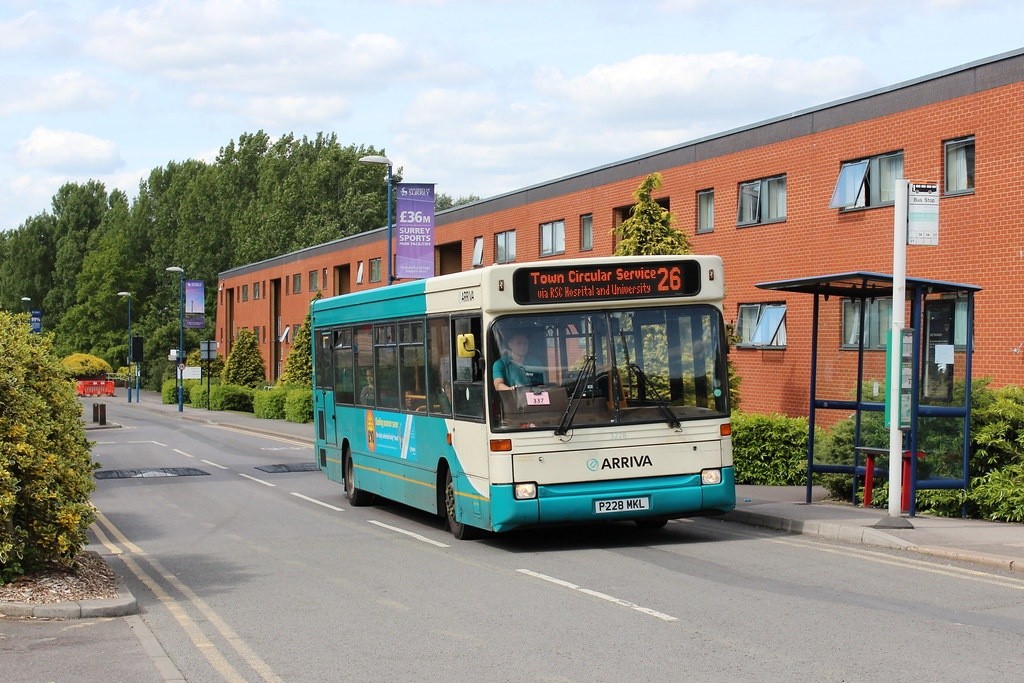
left=343, top=364, right=395, bottom=393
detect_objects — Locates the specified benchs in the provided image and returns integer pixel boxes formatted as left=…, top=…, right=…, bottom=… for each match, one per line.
left=852, top=446, right=927, bottom=509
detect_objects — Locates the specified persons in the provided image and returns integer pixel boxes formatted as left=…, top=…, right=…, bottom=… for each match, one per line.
left=360, top=367, right=375, bottom=406
left=493, top=333, right=547, bottom=392
left=405, top=389, right=416, bottom=410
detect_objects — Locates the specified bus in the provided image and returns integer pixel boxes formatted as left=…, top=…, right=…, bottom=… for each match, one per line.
left=306, top=248, right=739, bottom=544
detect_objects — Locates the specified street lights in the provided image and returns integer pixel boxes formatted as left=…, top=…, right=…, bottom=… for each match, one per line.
left=166, top=266, right=185, bottom=413
left=117, top=291, right=134, bottom=403
left=22, top=297, right=32, bottom=314
left=358, top=154, right=394, bottom=285
